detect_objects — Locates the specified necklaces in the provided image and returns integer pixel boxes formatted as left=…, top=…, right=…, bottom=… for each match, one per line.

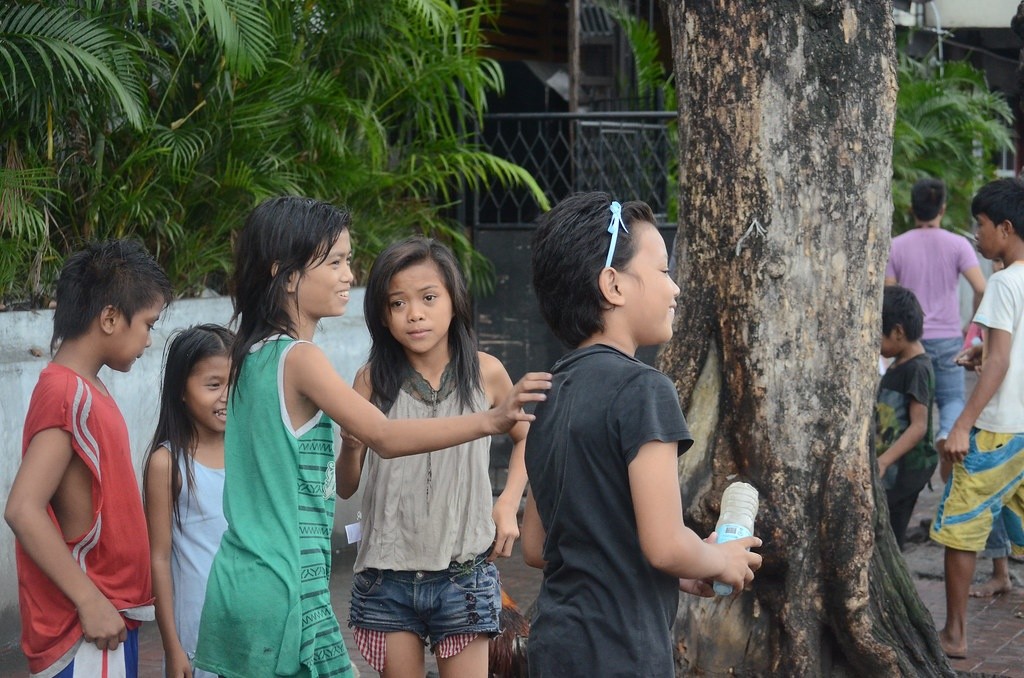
left=913, top=223, right=938, bottom=229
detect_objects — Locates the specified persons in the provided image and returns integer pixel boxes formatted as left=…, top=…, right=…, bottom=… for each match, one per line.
left=970, top=510, right=1011, bottom=601
left=936, top=178, right=1024, bottom=657
left=522, top=191, right=763, bottom=678
left=886, top=178, right=986, bottom=481
left=4, top=236, right=173, bottom=677
left=336, top=237, right=531, bottom=678
left=144, top=324, right=239, bottom=678
left=193, top=197, right=552, bottom=677
left=991, top=257, right=1005, bottom=273
left=873, top=286, right=938, bottom=548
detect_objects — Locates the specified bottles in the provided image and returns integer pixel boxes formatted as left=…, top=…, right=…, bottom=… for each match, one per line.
left=713, top=481, right=760, bottom=596
